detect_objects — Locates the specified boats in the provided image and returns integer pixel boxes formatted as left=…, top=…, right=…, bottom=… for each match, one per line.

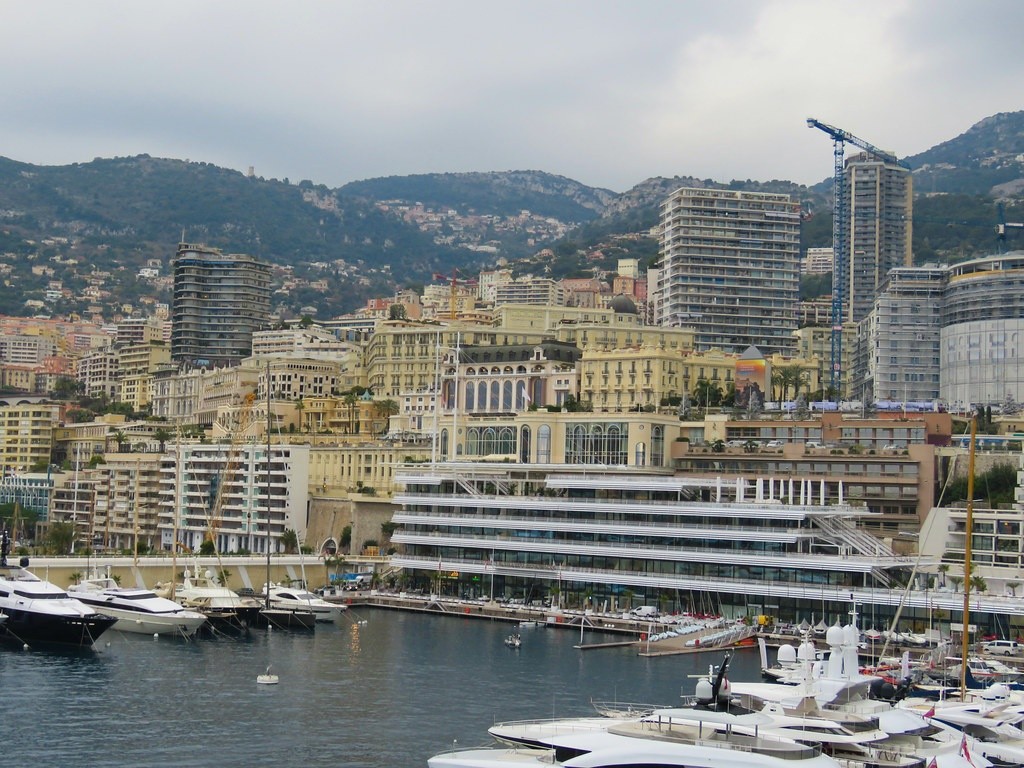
left=64, top=565, right=208, bottom=642
left=259, top=581, right=348, bottom=624
left=0, top=557, right=118, bottom=649
left=155, top=563, right=263, bottom=631
left=426, top=420, right=1024, bottom=768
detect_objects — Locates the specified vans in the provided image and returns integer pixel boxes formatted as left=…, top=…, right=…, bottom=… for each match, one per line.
left=982, top=641, right=1022, bottom=655
left=630, top=606, right=656, bottom=618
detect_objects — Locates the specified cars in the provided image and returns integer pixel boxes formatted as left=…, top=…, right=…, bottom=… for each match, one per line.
left=321, top=585, right=335, bottom=594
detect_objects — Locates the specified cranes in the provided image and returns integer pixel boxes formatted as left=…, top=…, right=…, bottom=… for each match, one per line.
left=835, top=200, right=1024, bottom=270
left=805, top=116, right=909, bottom=401
left=430, top=266, right=476, bottom=321
